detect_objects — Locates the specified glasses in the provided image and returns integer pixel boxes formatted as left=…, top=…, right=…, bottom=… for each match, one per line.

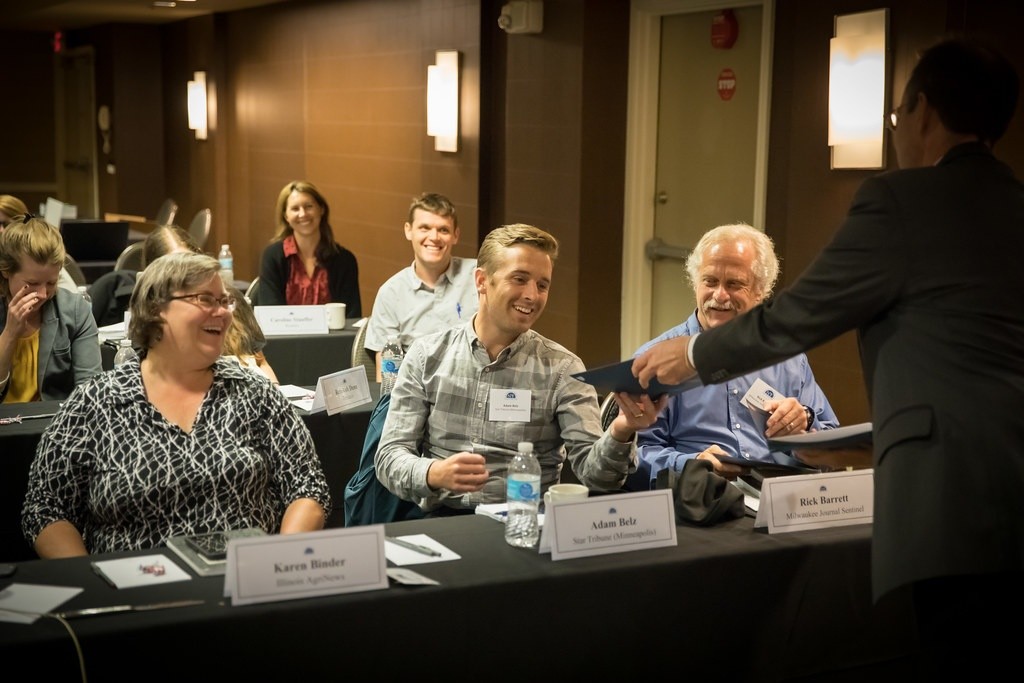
left=888, top=99, right=915, bottom=131
left=171, top=293, right=237, bottom=313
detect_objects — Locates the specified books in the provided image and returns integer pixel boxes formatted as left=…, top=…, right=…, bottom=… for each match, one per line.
left=472, top=501, right=546, bottom=530
left=165, top=527, right=270, bottom=576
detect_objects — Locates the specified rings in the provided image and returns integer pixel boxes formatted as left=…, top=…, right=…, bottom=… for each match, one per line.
left=789, top=422, right=794, bottom=429
left=634, top=414, right=643, bottom=418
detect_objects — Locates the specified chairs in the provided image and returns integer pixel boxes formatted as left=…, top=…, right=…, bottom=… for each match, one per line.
left=188, top=208, right=212, bottom=249
left=341, top=389, right=422, bottom=526
left=156, top=198, right=178, bottom=227
left=350, top=316, right=377, bottom=382
left=114, top=243, right=142, bottom=272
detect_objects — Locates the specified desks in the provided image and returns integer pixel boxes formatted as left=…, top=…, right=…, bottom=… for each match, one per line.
left=1, top=261, right=878, bottom=683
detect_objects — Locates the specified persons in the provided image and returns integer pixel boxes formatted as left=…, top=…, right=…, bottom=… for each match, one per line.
left=0, top=195, right=105, bottom=407
left=632, top=40, right=1024, bottom=683
left=19, top=250, right=329, bottom=562
left=373, top=223, right=668, bottom=520
left=364, top=193, right=479, bottom=382
left=633, top=224, right=839, bottom=489
left=143, top=226, right=279, bottom=385
left=256, top=180, right=362, bottom=319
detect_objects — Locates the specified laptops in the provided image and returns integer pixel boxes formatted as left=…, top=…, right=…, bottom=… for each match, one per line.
left=60, top=218, right=131, bottom=265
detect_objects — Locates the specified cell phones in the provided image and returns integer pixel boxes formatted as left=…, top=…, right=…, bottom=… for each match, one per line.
left=0, top=563, right=17, bottom=578
left=186, top=532, right=230, bottom=560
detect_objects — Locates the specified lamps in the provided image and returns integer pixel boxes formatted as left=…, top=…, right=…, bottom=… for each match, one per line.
left=426, top=50, right=462, bottom=153
left=828, top=9, right=893, bottom=172
left=187, top=71, right=208, bottom=140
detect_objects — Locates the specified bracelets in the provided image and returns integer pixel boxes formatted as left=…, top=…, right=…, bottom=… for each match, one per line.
left=0, top=370, right=10, bottom=385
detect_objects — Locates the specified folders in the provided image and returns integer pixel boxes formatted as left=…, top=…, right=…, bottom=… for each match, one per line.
left=569, top=359, right=704, bottom=400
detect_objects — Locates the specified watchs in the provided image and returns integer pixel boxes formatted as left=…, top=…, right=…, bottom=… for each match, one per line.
left=802, top=405, right=815, bottom=431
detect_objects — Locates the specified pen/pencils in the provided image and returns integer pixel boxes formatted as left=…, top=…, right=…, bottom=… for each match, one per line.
left=385, top=535, right=441, bottom=556
left=457, top=302, right=461, bottom=317
left=286, top=395, right=314, bottom=401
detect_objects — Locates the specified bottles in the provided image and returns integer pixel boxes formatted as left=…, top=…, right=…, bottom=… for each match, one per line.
left=380, top=334, right=403, bottom=398
left=504, top=441, right=542, bottom=548
left=114, top=340, right=136, bottom=365
left=218, top=244, right=233, bottom=288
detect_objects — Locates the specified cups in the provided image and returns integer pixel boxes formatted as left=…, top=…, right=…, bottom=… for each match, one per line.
left=543, top=481, right=591, bottom=503
left=325, top=303, right=346, bottom=329
